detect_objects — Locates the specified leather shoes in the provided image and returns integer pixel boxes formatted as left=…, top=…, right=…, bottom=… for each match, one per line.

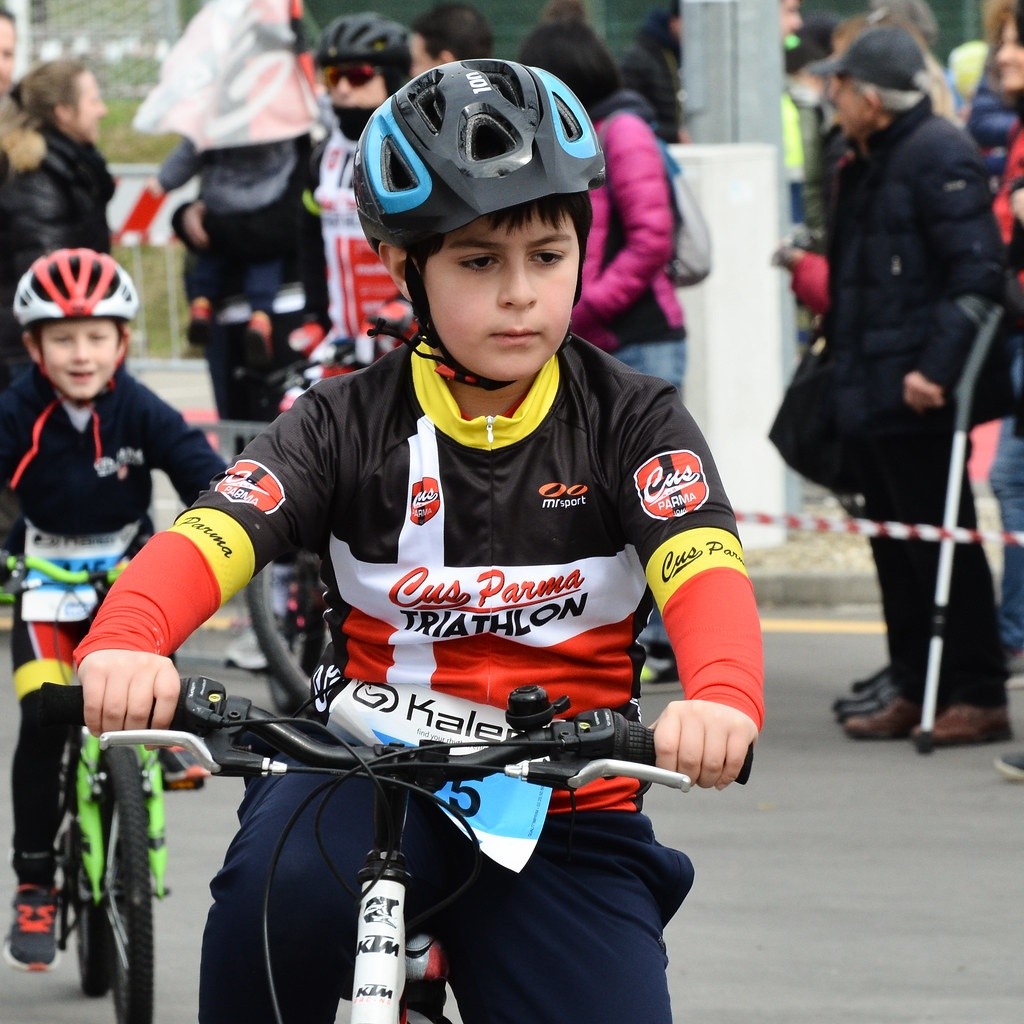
left=911, top=704, right=1012, bottom=747
left=843, top=696, right=923, bottom=741
left=831, top=672, right=900, bottom=727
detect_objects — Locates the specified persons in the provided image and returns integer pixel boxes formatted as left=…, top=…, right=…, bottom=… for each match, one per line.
left=772, top=0, right=1023, bottom=779
left=109, top=2, right=686, bottom=684
left=0, top=2, right=117, bottom=605
left=0, top=249, right=232, bottom=973
left=74, top=60, right=766, bottom=1024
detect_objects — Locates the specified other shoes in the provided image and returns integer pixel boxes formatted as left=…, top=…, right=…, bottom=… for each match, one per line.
left=244, top=318, right=272, bottom=367
left=185, top=306, right=213, bottom=348
left=851, top=662, right=893, bottom=694
left=994, top=752, right=1024, bottom=783
left=223, top=626, right=268, bottom=672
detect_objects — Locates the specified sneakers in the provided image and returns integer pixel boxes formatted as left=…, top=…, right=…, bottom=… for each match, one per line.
left=637, top=660, right=683, bottom=695
left=157, top=745, right=212, bottom=785
left=1, top=879, right=63, bottom=975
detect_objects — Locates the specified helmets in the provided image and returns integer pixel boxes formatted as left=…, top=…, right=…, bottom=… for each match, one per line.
left=352, top=58, right=608, bottom=255
left=315, top=12, right=413, bottom=68
left=11, top=246, right=141, bottom=328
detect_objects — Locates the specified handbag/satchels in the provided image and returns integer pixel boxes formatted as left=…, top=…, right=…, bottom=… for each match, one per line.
left=597, top=107, right=716, bottom=287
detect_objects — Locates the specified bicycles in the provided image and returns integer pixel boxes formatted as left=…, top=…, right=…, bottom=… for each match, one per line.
left=0, top=541, right=204, bottom=1024
left=98, top=681, right=754, bottom=1024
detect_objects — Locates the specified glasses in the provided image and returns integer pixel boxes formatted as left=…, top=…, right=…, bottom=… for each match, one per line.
left=322, top=61, right=384, bottom=90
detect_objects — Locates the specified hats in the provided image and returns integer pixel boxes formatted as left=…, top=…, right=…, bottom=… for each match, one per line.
left=806, top=24, right=928, bottom=90
